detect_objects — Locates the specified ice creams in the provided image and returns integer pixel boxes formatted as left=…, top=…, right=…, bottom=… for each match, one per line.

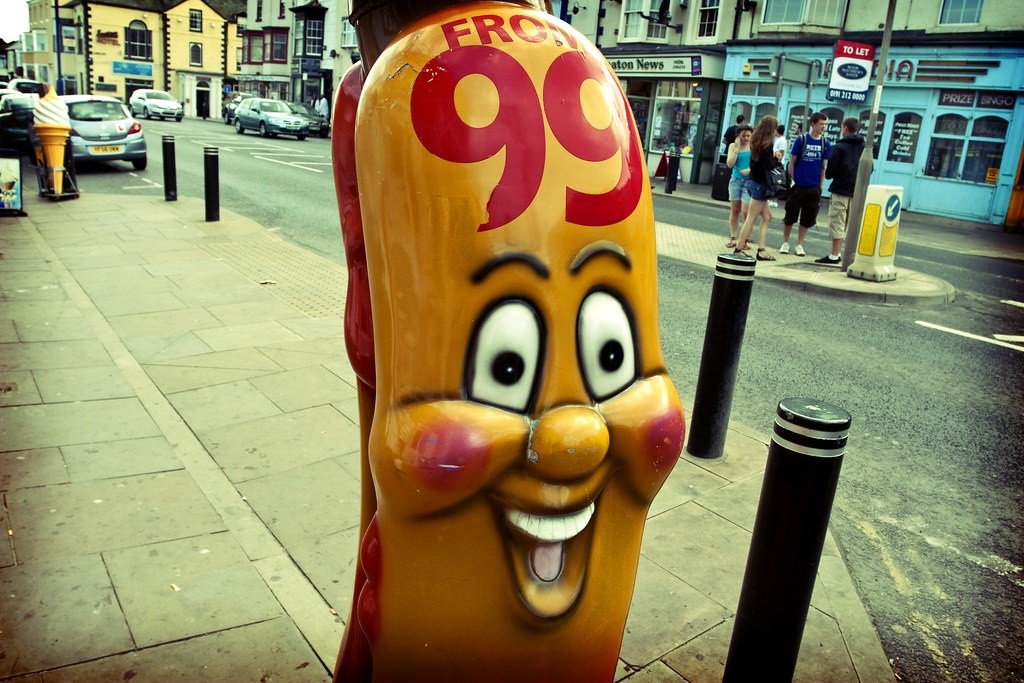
left=0, top=160, right=19, bottom=208
left=32, top=86, right=71, bottom=193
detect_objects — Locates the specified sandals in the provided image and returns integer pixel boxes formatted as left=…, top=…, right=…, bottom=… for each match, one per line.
left=726, top=237, right=737, bottom=248
left=743, top=240, right=751, bottom=250
left=733, top=248, right=754, bottom=260
left=757, top=248, right=776, bottom=261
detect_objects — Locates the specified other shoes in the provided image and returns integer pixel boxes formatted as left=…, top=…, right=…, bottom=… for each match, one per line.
left=794, top=245, right=805, bottom=255
left=780, top=242, right=789, bottom=253
left=815, top=256, right=841, bottom=264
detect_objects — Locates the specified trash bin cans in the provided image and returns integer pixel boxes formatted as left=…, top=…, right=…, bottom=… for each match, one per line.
left=710, top=162, right=731, bottom=202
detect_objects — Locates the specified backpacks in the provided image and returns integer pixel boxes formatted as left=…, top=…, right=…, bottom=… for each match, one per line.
left=836, top=140, right=861, bottom=189
left=786, top=135, right=826, bottom=189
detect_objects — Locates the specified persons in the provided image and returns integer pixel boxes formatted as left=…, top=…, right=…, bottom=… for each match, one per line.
left=722, top=115, right=745, bottom=155
left=310, top=94, right=329, bottom=122
left=780, top=113, right=831, bottom=256
left=733, top=115, right=782, bottom=260
left=815, top=118, right=874, bottom=264
left=727, top=123, right=752, bottom=250
left=794, top=123, right=803, bottom=137
left=767, top=125, right=787, bottom=207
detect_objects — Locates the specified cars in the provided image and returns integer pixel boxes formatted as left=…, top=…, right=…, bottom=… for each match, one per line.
left=128, top=89, right=184, bottom=123
left=233, top=97, right=310, bottom=142
left=0, top=73, right=45, bottom=147
left=222, top=91, right=262, bottom=125
left=55, top=94, right=149, bottom=172
left=285, top=102, right=329, bottom=138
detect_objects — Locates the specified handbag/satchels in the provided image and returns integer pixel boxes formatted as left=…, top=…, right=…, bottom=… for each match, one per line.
left=767, top=167, right=787, bottom=194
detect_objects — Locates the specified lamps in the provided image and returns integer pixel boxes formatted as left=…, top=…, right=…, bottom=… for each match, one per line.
left=741, top=0, right=756, bottom=11
left=162, top=135, right=175, bottom=142
left=203, top=146, right=219, bottom=155
left=573, top=1, right=586, bottom=14
left=714, top=254, right=757, bottom=282
left=330, top=50, right=339, bottom=58
left=771, top=397, right=852, bottom=457
